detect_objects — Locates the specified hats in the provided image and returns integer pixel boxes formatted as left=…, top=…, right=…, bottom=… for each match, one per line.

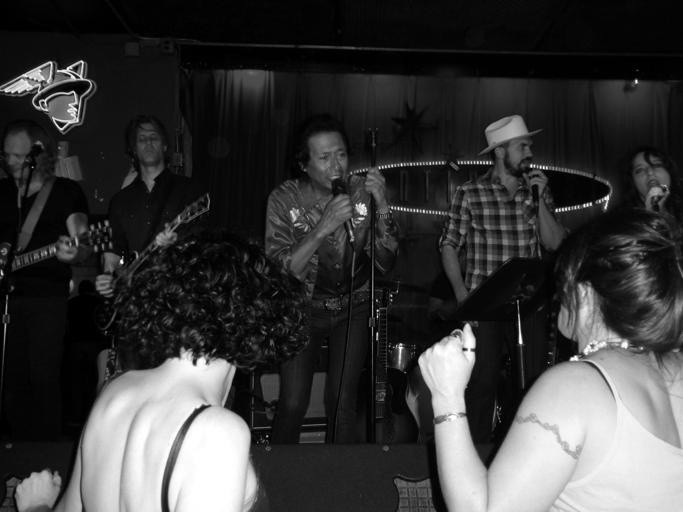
left=477, top=115, right=543, bottom=156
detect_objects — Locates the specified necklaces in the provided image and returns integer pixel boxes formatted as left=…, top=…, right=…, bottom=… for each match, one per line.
left=567, top=338, right=648, bottom=363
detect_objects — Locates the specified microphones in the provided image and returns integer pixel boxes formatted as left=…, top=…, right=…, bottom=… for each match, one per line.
left=23, top=139, right=45, bottom=167
left=649, top=183, right=669, bottom=208
left=526, top=168, right=545, bottom=212
left=329, top=177, right=360, bottom=247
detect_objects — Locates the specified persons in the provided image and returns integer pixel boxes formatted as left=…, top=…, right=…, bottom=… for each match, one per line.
left=13, top=237, right=308, bottom=512
left=0, top=119, right=89, bottom=440
left=93, top=113, right=203, bottom=300
left=601, top=145, right=683, bottom=230
left=417, top=208, right=683, bottom=512
left=437, top=114, right=566, bottom=424
left=262, top=114, right=399, bottom=446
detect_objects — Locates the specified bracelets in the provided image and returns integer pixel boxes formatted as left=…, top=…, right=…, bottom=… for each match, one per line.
left=432, top=412, right=468, bottom=425
left=376, top=205, right=391, bottom=214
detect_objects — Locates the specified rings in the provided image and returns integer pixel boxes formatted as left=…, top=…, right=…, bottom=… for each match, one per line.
left=462, top=348, right=476, bottom=353
left=449, top=329, right=463, bottom=343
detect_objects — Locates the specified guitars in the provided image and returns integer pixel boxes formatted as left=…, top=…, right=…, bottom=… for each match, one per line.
left=352, top=287, right=418, bottom=443
left=78, top=194, right=210, bottom=331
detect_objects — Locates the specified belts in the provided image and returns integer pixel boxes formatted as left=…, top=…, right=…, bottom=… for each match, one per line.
left=311, top=289, right=371, bottom=309
